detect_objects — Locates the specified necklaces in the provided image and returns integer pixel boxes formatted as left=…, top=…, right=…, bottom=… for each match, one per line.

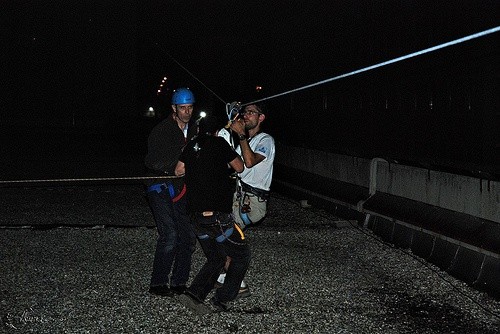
left=248, top=129, right=263, bottom=143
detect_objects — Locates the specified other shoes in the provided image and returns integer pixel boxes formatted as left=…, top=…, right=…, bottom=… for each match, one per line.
left=210, top=275, right=250, bottom=311
left=179, top=292, right=204, bottom=314
left=149, top=286, right=184, bottom=297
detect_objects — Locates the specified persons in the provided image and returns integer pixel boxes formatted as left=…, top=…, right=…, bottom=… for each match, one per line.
left=175, top=115, right=248, bottom=315
left=144, top=89, right=199, bottom=297
left=210, top=98, right=276, bottom=299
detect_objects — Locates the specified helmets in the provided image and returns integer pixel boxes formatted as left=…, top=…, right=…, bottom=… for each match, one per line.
left=171, top=88, right=195, bottom=104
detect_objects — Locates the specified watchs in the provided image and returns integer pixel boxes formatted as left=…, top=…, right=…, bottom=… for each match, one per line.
left=237, top=134, right=246, bottom=140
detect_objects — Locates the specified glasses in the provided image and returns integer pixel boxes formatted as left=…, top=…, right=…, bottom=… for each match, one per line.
left=240, top=111, right=262, bottom=116
